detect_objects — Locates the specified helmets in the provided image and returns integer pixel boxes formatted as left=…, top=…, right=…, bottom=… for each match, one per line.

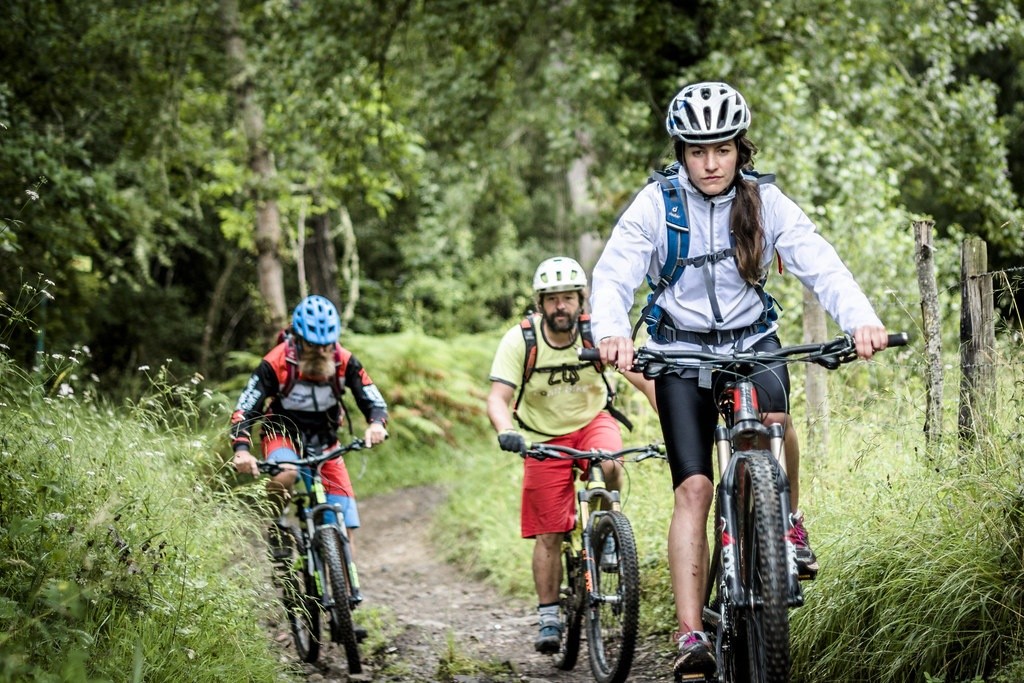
left=533, top=256, right=588, bottom=292
left=291, top=295, right=342, bottom=345
left=665, top=82, right=751, bottom=144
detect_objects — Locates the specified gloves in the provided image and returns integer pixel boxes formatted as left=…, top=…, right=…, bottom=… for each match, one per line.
left=497, top=429, right=527, bottom=458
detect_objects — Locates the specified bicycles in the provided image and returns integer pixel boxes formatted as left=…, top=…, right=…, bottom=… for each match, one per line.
left=232, top=433, right=389, bottom=674
left=519, top=442, right=668, bottom=683
left=577, top=332, right=909, bottom=683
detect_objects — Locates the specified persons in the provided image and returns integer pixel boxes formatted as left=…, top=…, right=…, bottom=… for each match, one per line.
left=230, top=295, right=388, bottom=640
left=588, top=81, right=888, bottom=683
left=487, top=256, right=658, bottom=655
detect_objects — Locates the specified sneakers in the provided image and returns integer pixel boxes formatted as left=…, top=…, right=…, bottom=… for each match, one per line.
left=788, top=515, right=819, bottom=572
left=601, top=534, right=621, bottom=574
left=266, top=527, right=293, bottom=565
left=673, top=633, right=717, bottom=683
left=535, top=615, right=564, bottom=654
